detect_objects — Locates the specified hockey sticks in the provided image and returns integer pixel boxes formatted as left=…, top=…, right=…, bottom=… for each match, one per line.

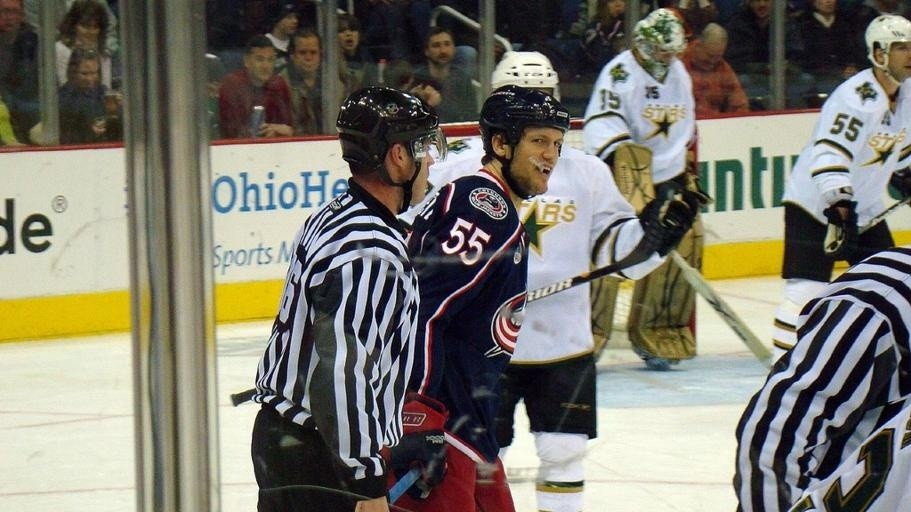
left=230, top=215, right=678, bottom=405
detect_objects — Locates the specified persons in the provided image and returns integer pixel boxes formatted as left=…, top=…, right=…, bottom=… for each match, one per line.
left=405, top=27, right=481, bottom=124
left=679, top=24, right=751, bottom=120
left=252, top=87, right=446, bottom=512
left=582, top=1, right=647, bottom=68
left=398, top=54, right=689, bottom=511
left=796, top=0, right=857, bottom=97
left=55, top=46, right=123, bottom=142
left=390, top=97, right=558, bottom=511
left=0, top=4, right=41, bottom=149
left=773, top=14, right=911, bottom=362
left=49, top=0, right=110, bottom=92
left=264, top=2, right=300, bottom=70
left=732, top=248, right=911, bottom=511
left=583, top=7, right=701, bottom=372
left=337, top=13, right=387, bottom=85
left=219, top=35, right=295, bottom=137
left=281, top=28, right=324, bottom=134
left=727, top=0, right=813, bottom=110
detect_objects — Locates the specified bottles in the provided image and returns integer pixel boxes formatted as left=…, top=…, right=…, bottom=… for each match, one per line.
left=251, top=105, right=267, bottom=137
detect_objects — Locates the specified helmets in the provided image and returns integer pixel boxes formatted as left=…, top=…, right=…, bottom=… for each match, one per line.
left=632, top=8, right=687, bottom=80
left=490, top=50, right=562, bottom=103
left=865, top=14, right=911, bottom=64
left=335, top=86, right=447, bottom=176
left=480, top=83, right=571, bottom=162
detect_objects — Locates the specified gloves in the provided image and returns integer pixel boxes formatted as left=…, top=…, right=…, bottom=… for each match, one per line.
left=637, top=185, right=708, bottom=261
left=391, top=389, right=449, bottom=507
left=823, top=200, right=861, bottom=257
